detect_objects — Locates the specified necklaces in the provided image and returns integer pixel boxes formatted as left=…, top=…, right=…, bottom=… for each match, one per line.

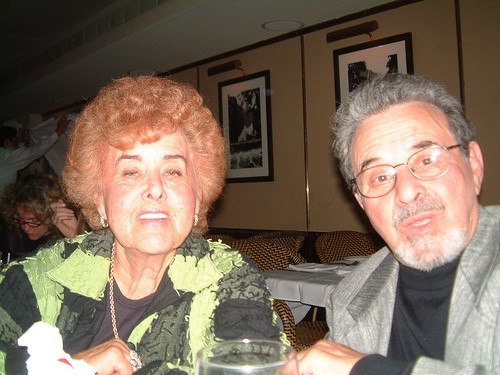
left=109, top=242, right=177, bottom=341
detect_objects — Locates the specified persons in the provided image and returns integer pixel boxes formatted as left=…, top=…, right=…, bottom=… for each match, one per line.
left=278, top=74, right=500, bottom=375
left=0, top=75, right=294, bottom=375
left=0, top=113, right=82, bottom=261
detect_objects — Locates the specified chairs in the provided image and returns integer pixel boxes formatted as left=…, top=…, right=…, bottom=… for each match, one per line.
left=204, top=231, right=385, bottom=352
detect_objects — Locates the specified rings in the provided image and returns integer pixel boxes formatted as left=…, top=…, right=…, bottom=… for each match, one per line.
left=130, top=349, right=143, bottom=372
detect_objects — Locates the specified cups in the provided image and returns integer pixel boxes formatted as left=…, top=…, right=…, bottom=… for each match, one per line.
left=195, top=339, right=299, bottom=375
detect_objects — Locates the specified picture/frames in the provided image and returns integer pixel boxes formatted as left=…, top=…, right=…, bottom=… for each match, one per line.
left=218, top=70, right=274, bottom=183
left=332, top=32, right=414, bottom=112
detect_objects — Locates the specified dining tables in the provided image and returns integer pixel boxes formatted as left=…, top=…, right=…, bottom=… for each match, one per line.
left=263, top=254, right=370, bottom=326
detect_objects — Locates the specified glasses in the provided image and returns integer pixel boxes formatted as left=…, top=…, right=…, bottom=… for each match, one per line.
left=17, top=218, right=45, bottom=228
left=348, top=140, right=469, bottom=198
